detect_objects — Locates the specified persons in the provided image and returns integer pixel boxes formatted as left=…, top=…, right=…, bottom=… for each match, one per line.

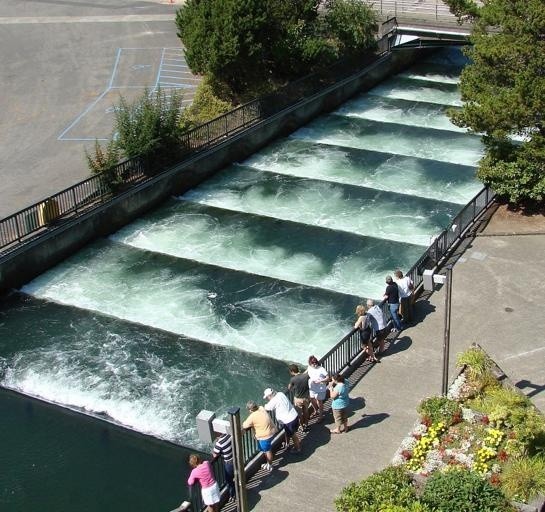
left=242, top=399, right=280, bottom=471
left=187, top=454, right=221, bottom=512
left=262, top=386, right=304, bottom=454
left=212, top=432, right=237, bottom=502
left=287, top=364, right=310, bottom=431
left=325, top=373, right=351, bottom=434
left=306, top=355, right=329, bottom=424
left=394, top=270, right=415, bottom=324
left=353, top=305, right=380, bottom=363
left=366, top=299, right=390, bottom=355
left=383, top=275, right=403, bottom=332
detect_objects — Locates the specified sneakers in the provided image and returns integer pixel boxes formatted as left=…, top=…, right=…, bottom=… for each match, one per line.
left=260, top=463, right=273, bottom=471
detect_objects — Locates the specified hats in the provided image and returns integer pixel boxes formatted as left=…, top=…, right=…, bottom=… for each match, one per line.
left=263, top=388, right=273, bottom=398
left=366, top=298, right=373, bottom=305
left=385, top=276, right=393, bottom=283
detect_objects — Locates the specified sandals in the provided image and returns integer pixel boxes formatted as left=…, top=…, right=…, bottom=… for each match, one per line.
left=309, top=412, right=323, bottom=421
left=369, top=347, right=387, bottom=363
left=281, top=441, right=302, bottom=452
left=331, top=426, right=349, bottom=433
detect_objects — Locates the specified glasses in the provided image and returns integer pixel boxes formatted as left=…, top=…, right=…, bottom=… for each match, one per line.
left=310, top=361, right=317, bottom=364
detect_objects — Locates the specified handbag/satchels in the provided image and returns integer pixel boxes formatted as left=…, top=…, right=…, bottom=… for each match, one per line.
left=361, top=329, right=370, bottom=342
